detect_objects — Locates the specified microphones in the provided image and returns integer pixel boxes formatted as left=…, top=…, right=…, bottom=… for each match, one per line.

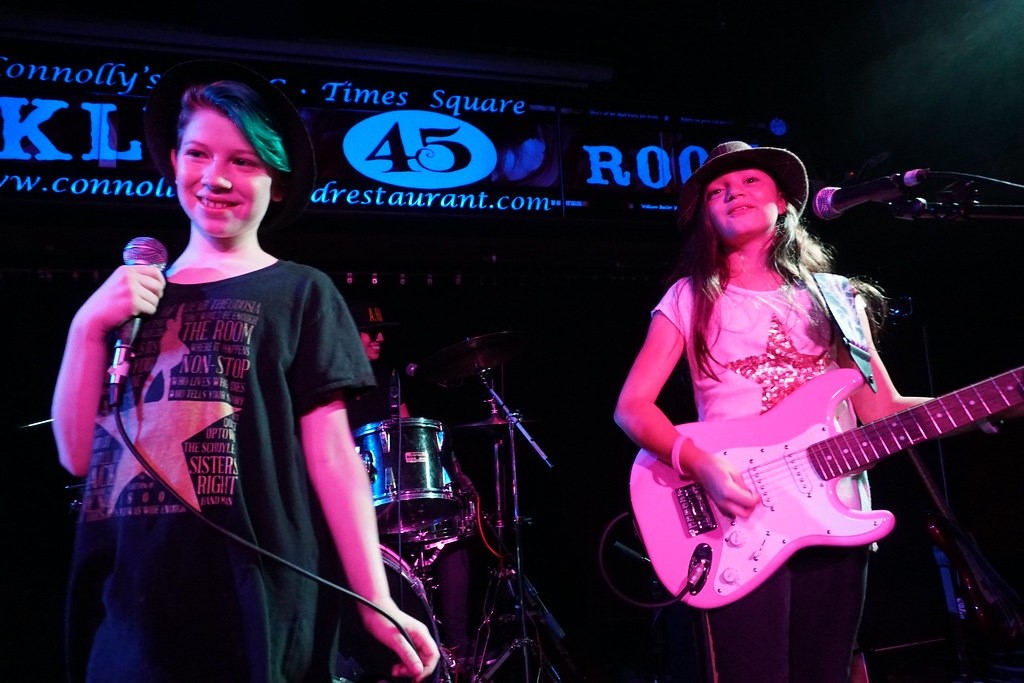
left=388, top=371, right=398, bottom=408
left=811, top=169, right=930, bottom=220
left=110, top=236, right=168, bottom=408
left=407, top=362, right=449, bottom=389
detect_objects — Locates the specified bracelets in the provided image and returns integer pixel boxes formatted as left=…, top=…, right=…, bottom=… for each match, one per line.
left=672, top=436, right=693, bottom=477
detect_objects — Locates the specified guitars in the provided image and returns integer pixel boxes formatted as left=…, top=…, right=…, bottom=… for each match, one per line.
left=906, top=446, right=1024, bottom=645
left=625, top=363, right=1024, bottom=612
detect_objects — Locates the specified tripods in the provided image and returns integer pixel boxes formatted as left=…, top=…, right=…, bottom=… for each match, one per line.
left=469, top=361, right=589, bottom=683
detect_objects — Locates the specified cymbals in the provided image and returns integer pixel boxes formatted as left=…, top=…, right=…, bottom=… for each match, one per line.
left=451, top=408, right=541, bottom=432
left=415, top=328, right=534, bottom=382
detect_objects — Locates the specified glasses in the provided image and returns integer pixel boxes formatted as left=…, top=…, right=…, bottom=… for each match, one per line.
left=359, top=322, right=384, bottom=336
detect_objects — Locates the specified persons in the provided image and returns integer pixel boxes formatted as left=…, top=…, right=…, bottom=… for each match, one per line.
left=351, top=300, right=470, bottom=655
left=49, top=79, right=440, bottom=683
left=612, top=140, right=998, bottom=683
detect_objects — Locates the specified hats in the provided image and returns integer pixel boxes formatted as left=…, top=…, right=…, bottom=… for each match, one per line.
left=677, top=138, right=809, bottom=228
left=145, top=58, right=317, bottom=231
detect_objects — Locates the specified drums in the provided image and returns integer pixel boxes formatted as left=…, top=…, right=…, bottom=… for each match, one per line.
left=390, top=479, right=477, bottom=545
left=330, top=540, right=455, bottom=682
left=351, top=416, right=465, bottom=538
